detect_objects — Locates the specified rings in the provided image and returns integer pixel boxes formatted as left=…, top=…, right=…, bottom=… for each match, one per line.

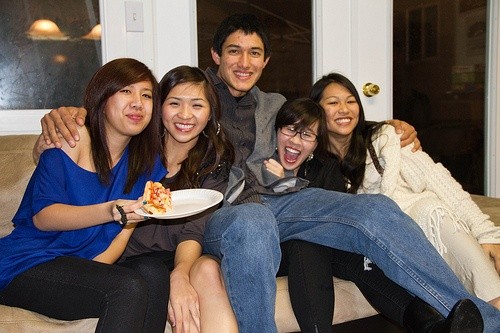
left=116, top=205, right=128, bottom=225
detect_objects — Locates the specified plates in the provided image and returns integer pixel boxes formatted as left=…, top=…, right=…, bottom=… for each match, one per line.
left=134, top=188, right=223, bottom=219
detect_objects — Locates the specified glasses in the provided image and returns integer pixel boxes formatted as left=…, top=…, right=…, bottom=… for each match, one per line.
left=280, top=126, right=318, bottom=142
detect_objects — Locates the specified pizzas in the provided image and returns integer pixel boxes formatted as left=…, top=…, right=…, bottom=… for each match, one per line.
left=141, top=181, right=172, bottom=214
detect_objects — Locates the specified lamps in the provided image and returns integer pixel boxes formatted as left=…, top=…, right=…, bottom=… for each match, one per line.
left=25, top=19, right=66, bottom=40
left=81, top=24, right=101, bottom=40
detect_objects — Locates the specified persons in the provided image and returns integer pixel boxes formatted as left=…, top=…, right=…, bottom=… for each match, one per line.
left=264, top=97, right=485, bottom=333
left=0, top=58, right=170, bottom=333
left=41, top=13, right=500, bottom=333
left=32, top=66, right=239, bottom=333
left=309, top=73, right=500, bottom=311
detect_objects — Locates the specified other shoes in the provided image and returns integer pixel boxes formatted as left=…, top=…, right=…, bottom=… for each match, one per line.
left=445, top=298, right=484, bottom=333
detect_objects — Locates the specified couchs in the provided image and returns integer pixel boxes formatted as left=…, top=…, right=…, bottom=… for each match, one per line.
left=0, top=135, right=381, bottom=333
